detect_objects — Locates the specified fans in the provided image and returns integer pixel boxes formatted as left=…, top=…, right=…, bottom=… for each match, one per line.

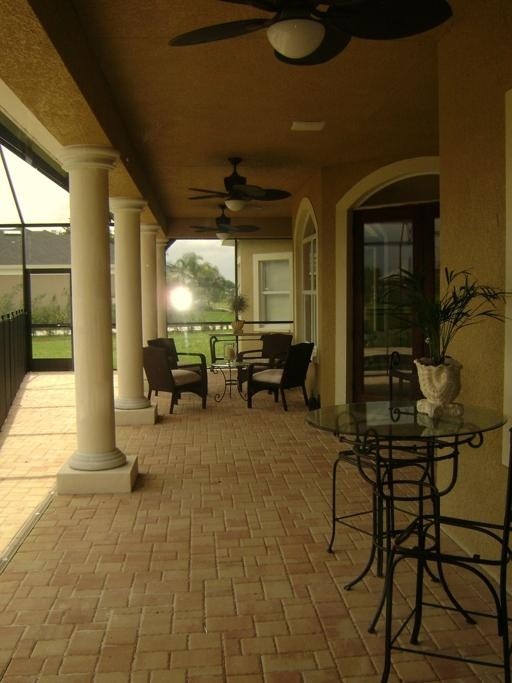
left=185, top=157, right=292, bottom=239
left=168, top=0, right=453, bottom=66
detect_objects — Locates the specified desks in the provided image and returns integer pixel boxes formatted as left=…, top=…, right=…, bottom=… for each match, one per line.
left=206, top=331, right=294, bottom=373
left=300, top=398, right=507, bottom=636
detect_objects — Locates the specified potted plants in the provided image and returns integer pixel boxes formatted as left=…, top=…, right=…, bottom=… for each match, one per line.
left=226, top=293, right=247, bottom=335
left=374, top=264, right=511, bottom=420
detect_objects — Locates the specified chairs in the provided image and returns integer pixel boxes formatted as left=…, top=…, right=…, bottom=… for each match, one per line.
left=377, top=427, right=511, bottom=683
left=142, top=334, right=314, bottom=413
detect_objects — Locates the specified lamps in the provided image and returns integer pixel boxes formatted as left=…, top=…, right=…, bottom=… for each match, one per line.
left=267, top=10, right=326, bottom=60
